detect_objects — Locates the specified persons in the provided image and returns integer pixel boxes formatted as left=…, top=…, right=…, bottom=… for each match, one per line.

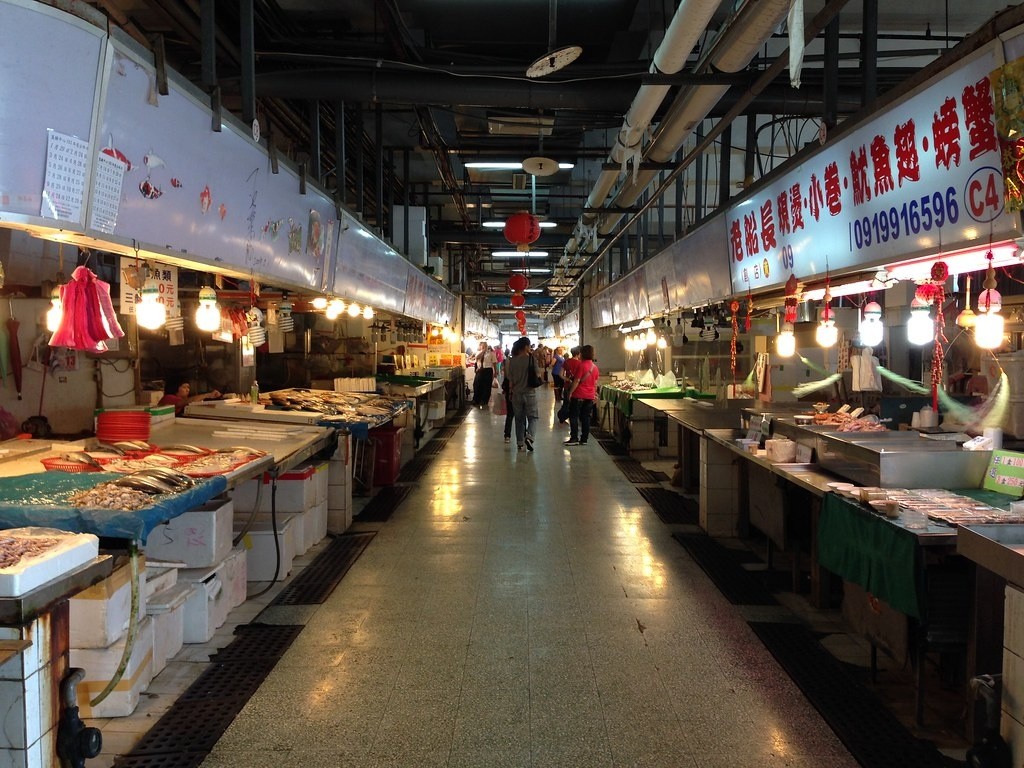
left=508, top=337, right=542, bottom=452
left=156, top=375, right=222, bottom=416
left=467, top=344, right=511, bottom=388
left=563, top=344, right=600, bottom=446
left=557, top=347, right=582, bottom=425
left=474, top=342, right=498, bottom=408
left=530, top=343, right=574, bottom=385
left=550, top=346, right=563, bottom=400
left=467, top=342, right=484, bottom=404
left=503, top=347, right=527, bottom=444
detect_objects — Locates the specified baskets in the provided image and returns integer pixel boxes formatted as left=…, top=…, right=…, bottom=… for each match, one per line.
left=41, top=446, right=256, bottom=478
left=96, top=411, right=152, bottom=444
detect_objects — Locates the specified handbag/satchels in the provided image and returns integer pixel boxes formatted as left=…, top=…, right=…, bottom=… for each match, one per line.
left=848, top=347, right=883, bottom=392
left=492, top=377, right=500, bottom=388
left=528, top=352, right=543, bottom=388
left=493, top=394, right=507, bottom=415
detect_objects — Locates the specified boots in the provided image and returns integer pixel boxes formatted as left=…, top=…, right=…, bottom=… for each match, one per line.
left=554, top=388, right=560, bottom=402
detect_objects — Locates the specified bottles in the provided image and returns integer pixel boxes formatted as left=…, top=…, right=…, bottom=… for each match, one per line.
left=251, top=380, right=259, bottom=405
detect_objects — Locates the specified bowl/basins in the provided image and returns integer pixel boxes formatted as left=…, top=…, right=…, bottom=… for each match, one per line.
left=97, top=412, right=150, bottom=444
left=826, top=481, right=854, bottom=494
left=868, top=499, right=887, bottom=514
left=850, top=488, right=860, bottom=500
left=837, top=485, right=857, bottom=499
left=793, top=415, right=815, bottom=425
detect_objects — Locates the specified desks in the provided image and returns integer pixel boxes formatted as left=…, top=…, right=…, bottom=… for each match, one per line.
left=600, top=380, right=1024, bottom=768
left=0, top=370, right=453, bottom=543
left=0, top=558, right=120, bottom=768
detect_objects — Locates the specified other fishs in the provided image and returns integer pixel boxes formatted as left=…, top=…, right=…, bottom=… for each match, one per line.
left=61, top=440, right=262, bottom=494
left=269, top=388, right=405, bottom=426
left=101, top=133, right=226, bottom=222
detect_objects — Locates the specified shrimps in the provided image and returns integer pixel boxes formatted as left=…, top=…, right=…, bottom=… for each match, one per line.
left=0, top=534, right=61, bottom=567
left=68, top=485, right=153, bottom=510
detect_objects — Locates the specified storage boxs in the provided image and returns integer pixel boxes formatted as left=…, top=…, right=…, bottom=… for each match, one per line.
left=68, top=461, right=328, bottom=719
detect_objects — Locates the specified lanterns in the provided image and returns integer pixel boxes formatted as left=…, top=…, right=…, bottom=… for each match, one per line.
left=913, top=279, right=941, bottom=308
left=510, top=290, right=527, bottom=308
left=503, top=209, right=542, bottom=252
left=514, top=310, right=527, bottom=336
left=508, top=272, right=528, bottom=296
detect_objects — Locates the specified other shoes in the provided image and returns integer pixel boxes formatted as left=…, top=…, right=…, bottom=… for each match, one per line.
left=525, top=439, right=534, bottom=451
left=505, top=437, right=510, bottom=443
left=579, top=441, right=587, bottom=444
left=518, top=445, right=524, bottom=449
left=563, top=439, right=579, bottom=446
